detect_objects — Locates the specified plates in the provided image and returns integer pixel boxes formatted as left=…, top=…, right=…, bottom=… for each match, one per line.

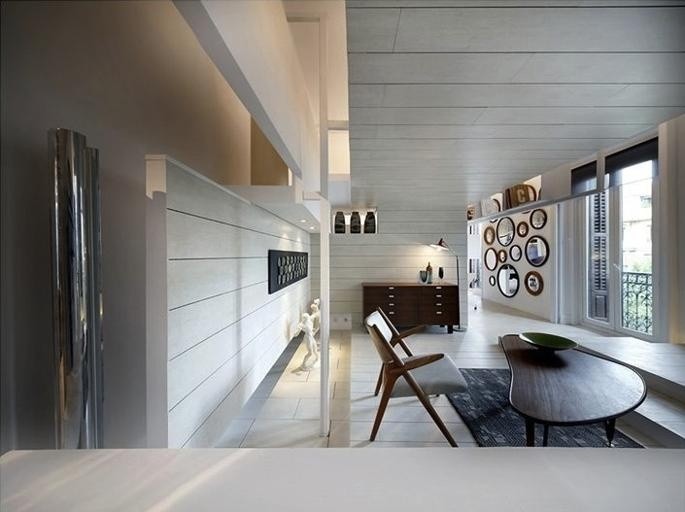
left=518, top=332, right=578, bottom=355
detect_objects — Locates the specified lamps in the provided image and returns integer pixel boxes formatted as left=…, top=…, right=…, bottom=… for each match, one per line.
left=429, top=237, right=467, bottom=333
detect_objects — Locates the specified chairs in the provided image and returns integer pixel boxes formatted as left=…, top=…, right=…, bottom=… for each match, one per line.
left=363, top=306, right=468, bottom=447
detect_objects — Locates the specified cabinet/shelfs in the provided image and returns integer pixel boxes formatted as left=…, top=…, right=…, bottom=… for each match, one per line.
left=361, top=282, right=459, bottom=334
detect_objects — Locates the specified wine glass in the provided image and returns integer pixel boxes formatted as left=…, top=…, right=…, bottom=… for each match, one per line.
left=438, top=267, right=444, bottom=284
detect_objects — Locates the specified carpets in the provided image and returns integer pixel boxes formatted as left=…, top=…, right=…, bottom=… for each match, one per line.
left=446, top=367, right=645, bottom=448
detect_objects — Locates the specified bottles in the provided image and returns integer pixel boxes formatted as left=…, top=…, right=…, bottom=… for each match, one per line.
left=334, top=212, right=375, bottom=234
left=426, top=262, right=433, bottom=284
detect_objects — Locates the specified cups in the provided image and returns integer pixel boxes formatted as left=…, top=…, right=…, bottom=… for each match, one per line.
left=420, top=271, right=427, bottom=282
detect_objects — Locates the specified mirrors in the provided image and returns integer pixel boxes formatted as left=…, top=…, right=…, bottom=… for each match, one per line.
left=484, top=209, right=549, bottom=298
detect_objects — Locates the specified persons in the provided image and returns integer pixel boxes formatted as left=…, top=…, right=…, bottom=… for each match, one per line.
left=298, top=297, right=320, bottom=371
